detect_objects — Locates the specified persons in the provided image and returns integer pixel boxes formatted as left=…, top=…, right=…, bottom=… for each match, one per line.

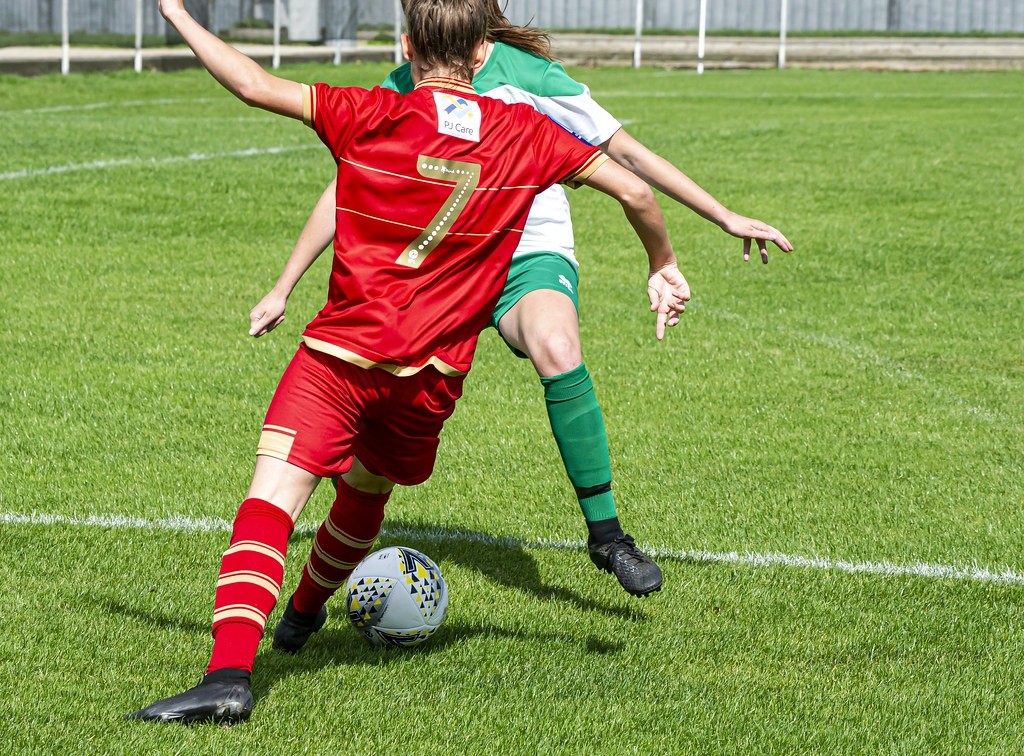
left=130, top=1, right=692, bottom=725
left=248, top=1, right=792, bottom=599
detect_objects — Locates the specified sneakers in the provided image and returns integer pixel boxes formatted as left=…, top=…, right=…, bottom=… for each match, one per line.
left=125, top=679, right=254, bottom=727
left=588, top=533, right=663, bottom=598
left=273, top=596, right=328, bottom=652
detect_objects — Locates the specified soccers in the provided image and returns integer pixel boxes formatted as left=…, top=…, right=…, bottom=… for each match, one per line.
left=344, top=544, right=451, bottom=651
